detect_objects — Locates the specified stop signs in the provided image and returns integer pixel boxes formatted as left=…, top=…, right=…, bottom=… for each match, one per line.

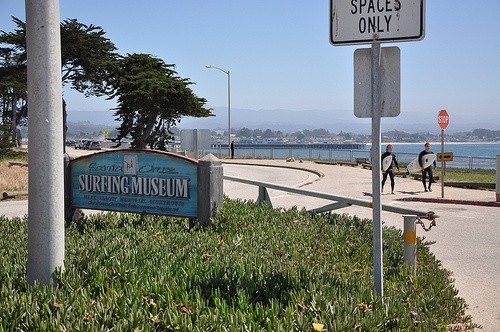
left=437, top=110, right=449, bottom=197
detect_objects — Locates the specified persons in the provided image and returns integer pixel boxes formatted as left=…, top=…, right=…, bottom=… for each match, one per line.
left=418, top=142, right=437, bottom=192
left=231, top=141, right=235, bottom=159
left=381, top=144, right=400, bottom=194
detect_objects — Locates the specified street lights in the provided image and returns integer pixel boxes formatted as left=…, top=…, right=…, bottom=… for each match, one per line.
left=205, top=65, right=230, bottom=159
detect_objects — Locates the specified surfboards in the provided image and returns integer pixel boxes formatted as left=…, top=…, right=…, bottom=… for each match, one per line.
left=407, top=154, right=437, bottom=174
left=383, top=156, right=392, bottom=171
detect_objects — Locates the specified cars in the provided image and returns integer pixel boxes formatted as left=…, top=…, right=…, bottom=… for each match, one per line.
left=66, top=138, right=101, bottom=150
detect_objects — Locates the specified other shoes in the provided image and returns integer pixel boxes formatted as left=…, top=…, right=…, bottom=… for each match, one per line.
left=424, top=190, right=428, bottom=192
left=428, top=187, right=432, bottom=192
